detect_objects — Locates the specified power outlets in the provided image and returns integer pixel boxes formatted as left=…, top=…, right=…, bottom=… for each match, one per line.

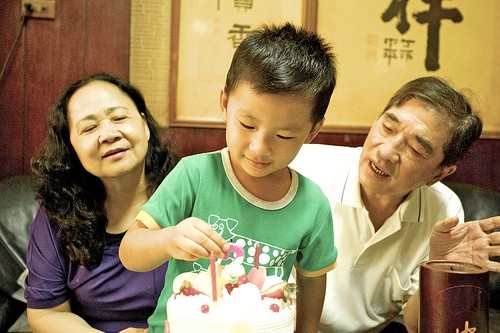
left=20, top=0, right=56, bottom=19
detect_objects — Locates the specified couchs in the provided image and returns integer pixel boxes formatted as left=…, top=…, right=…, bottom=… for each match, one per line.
left=0, top=176, right=500, bottom=333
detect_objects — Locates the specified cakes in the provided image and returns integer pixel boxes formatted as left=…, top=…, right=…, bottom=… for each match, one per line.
left=166, top=242, right=296, bottom=333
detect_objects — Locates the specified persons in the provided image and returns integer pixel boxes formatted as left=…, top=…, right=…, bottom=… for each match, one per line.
left=24, top=72, right=183, bottom=333
left=289, top=77, right=500, bottom=333
left=118, top=21, right=337, bottom=333
